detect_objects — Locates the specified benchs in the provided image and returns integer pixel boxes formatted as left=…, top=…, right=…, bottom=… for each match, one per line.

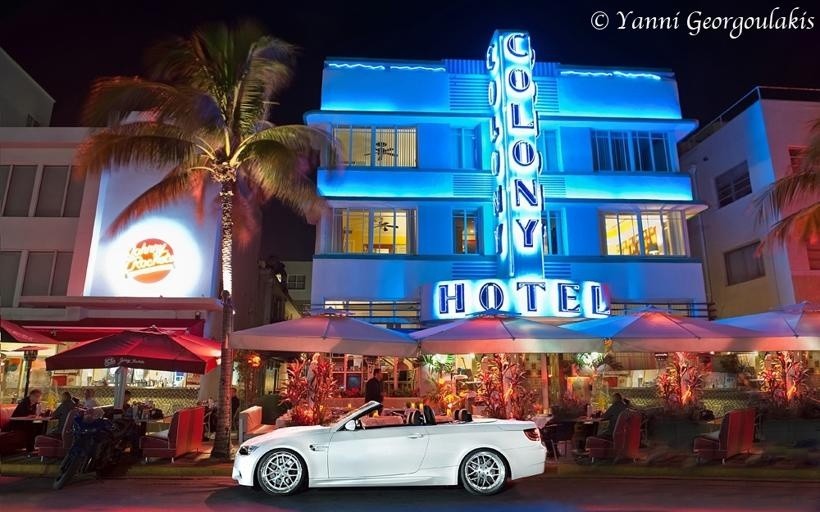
left=0, top=357, right=782, bottom=474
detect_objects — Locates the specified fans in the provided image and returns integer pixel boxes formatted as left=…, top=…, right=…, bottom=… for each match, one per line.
left=372, top=217, right=400, bottom=233
left=364, top=139, right=401, bottom=162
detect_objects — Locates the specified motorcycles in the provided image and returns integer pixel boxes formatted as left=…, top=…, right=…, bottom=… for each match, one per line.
left=51, top=408, right=146, bottom=492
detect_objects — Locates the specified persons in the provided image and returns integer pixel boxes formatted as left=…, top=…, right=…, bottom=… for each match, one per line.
left=210, top=386, right=239, bottom=435
left=5, top=390, right=130, bottom=459
left=594, top=392, right=631, bottom=441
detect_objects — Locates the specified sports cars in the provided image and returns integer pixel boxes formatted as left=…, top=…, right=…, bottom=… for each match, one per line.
left=228, top=398, right=551, bottom=498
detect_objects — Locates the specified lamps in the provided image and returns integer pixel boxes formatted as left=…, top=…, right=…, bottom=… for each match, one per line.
left=646, top=242, right=658, bottom=256
